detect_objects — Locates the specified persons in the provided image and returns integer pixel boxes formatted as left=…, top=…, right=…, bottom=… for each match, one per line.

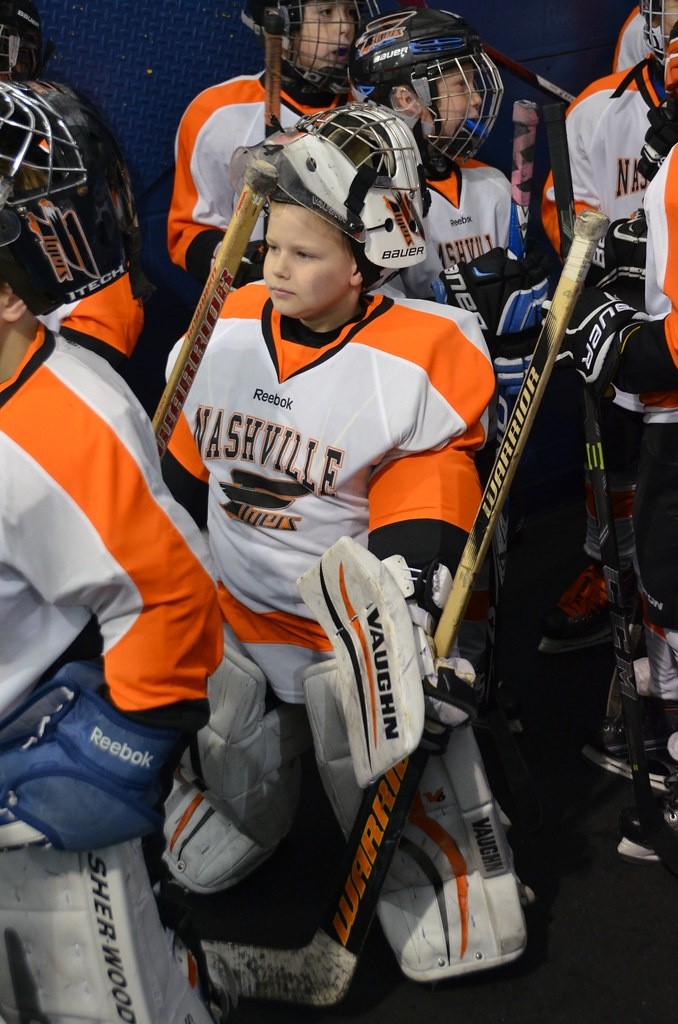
left=154, top=188, right=497, bottom=913
left=168, top=2, right=357, bottom=293
left=0, top=2, right=145, bottom=362
left=347, top=9, right=547, bottom=745
left=574, top=22, right=677, bottom=860
left=0, top=156, right=237, bottom=1024
left=539, top=0, right=678, bottom=637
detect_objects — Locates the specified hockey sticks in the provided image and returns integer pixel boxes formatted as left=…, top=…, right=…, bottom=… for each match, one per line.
left=499, top=99, right=540, bottom=297
left=264, top=17, right=281, bottom=256
left=147, top=158, right=280, bottom=459
left=398, top=0, right=576, bottom=102
left=202, top=210, right=617, bottom=1008
left=541, top=105, right=678, bottom=880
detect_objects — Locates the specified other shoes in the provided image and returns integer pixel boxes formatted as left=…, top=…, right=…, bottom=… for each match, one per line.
left=600, top=696, right=670, bottom=760
left=614, top=819, right=676, bottom=865
left=582, top=725, right=672, bottom=793
left=554, top=563, right=612, bottom=634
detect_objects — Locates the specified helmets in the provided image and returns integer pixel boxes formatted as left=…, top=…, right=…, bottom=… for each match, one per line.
left=0, top=0, right=45, bottom=83
left=2, top=79, right=146, bottom=319
left=229, top=102, right=430, bottom=268
left=348, top=8, right=506, bottom=165
left=637, top=0, right=678, bottom=69
left=241, top=0, right=384, bottom=99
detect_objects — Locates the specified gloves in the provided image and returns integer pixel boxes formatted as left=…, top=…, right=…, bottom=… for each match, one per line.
left=608, top=317, right=677, bottom=394
left=0, top=658, right=179, bottom=854
left=430, top=241, right=552, bottom=332
left=488, top=338, right=540, bottom=398
